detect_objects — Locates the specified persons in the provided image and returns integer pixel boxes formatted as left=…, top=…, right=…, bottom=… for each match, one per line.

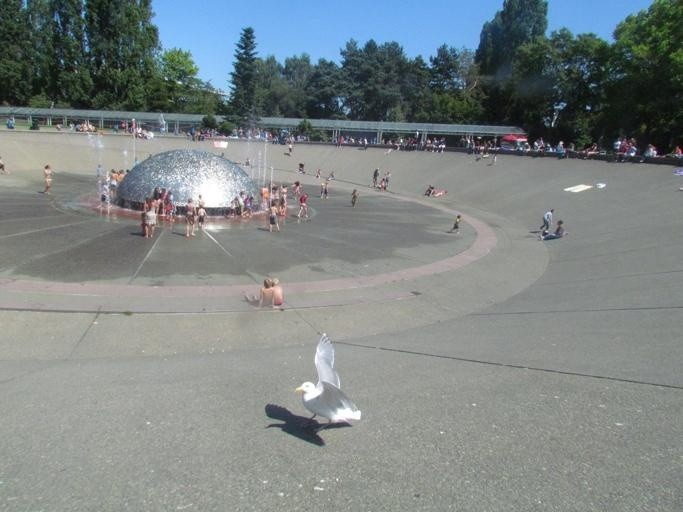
left=318, top=134, right=323, bottom=142
left=56, top=120, right=96, bottom=132
left=331, top=171, right=335, bottom=180
left=271, top=278, right=284, bottom=305
left=432, top=189, right=446, bottom=197
left=539, top=208, right=553, bottom=232
left=385, top=172, right=390, bottom=189
left=539, top=219, right=564, bottom=240
left=112, top=118, right=309, bottom=144
left=96, top=158, right=310, bottom=238
left=372, top=169, right=379, bottom=187
left=447, top=214, right=461, bottom=234
left=423, top=184, right=434, bottom=198
left=44, top=165, right=54, bottom=194
left=0, top=156, right=10, bottom=174
left=319, top=183, right=323, bottom=198
left=286, top=143, right=292, bottom=156
left=351, top=190, right=357, bottom=208
left=376, top=179, right=385, bottom=190
left=314, top=169, right=320, bottom=179
left=244, top=278, right=276, bottom=310
left=324, top=182, right=329, bottom=199
left=326, top=173, right=331, bottom=181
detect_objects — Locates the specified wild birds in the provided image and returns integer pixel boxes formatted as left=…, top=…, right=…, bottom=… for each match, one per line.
left=294, top=332, right=362, bottom=433
